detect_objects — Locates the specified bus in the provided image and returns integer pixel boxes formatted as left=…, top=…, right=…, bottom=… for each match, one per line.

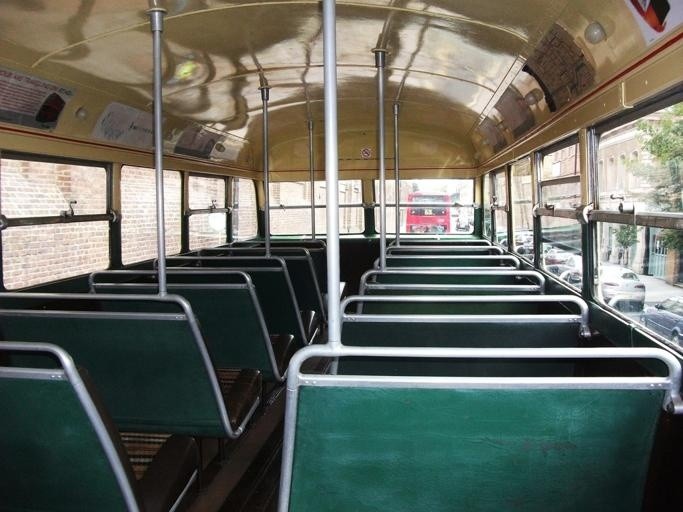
left=405, top=191, right=450, bottom=233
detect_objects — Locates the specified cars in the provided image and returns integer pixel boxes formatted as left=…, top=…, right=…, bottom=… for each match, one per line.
left=456, top=212, right=682, bottom=339
